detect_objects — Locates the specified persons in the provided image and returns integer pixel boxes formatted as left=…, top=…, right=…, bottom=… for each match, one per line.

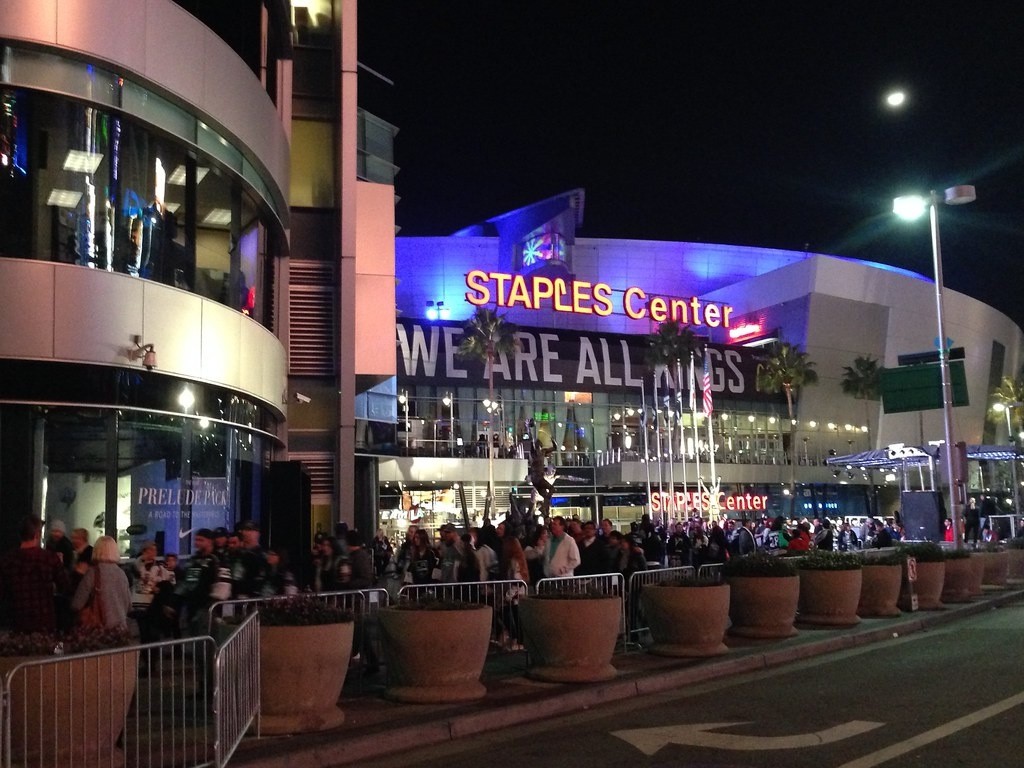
left=527, top=417, right=558, bottom=519
left=0, top=488, right=1024, bottom=700
left=477, top=433, right=524, bottom=459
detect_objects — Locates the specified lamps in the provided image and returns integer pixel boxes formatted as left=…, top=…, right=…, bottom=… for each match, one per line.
left=129, top=342, right=159, bottom=372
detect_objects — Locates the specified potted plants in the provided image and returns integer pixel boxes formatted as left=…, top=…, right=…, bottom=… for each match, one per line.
left=856, top=553, right=904, bottom=618
left=640, top=576, right=732, bottom=656
left=1004, top=537, right=1024, bottom=584
left=893, top=541, right=947, bottom=610
left=796, top=546, right=863, bottom=625
left=371, top=591, right=493, bottom=705
left=218, top=591, right=356, bottom=735
left=0, top=619, right=142, bottom=768
left=723, top=548, right=803, bottom=638
left=944, top=548, right=985, bottom=603
left=973, top=542, right=1010, bottom=586
left=513, top=584, right=623, bottom=683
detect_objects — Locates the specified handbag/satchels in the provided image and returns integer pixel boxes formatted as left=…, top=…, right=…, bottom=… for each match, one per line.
left=81, top=566, right=107, bottom=632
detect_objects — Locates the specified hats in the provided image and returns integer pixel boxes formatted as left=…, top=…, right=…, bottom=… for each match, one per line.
left=437, top=525, right=447, bottom=531
left=212, top=527, right=228, bottom=539
left=233, top=521, right=260, bottom=531
left=444, top=523, right=455, bottom=531
left=50, top=520, right=66, bottom=533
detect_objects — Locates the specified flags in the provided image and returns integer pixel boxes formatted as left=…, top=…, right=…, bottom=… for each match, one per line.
left=639, top=345, right=714, bottom=430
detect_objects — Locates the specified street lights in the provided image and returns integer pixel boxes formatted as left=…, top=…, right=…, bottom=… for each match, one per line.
left=992, top=402, right=1024, bottom=535
left=888, top=184, right=978, bottom=557
